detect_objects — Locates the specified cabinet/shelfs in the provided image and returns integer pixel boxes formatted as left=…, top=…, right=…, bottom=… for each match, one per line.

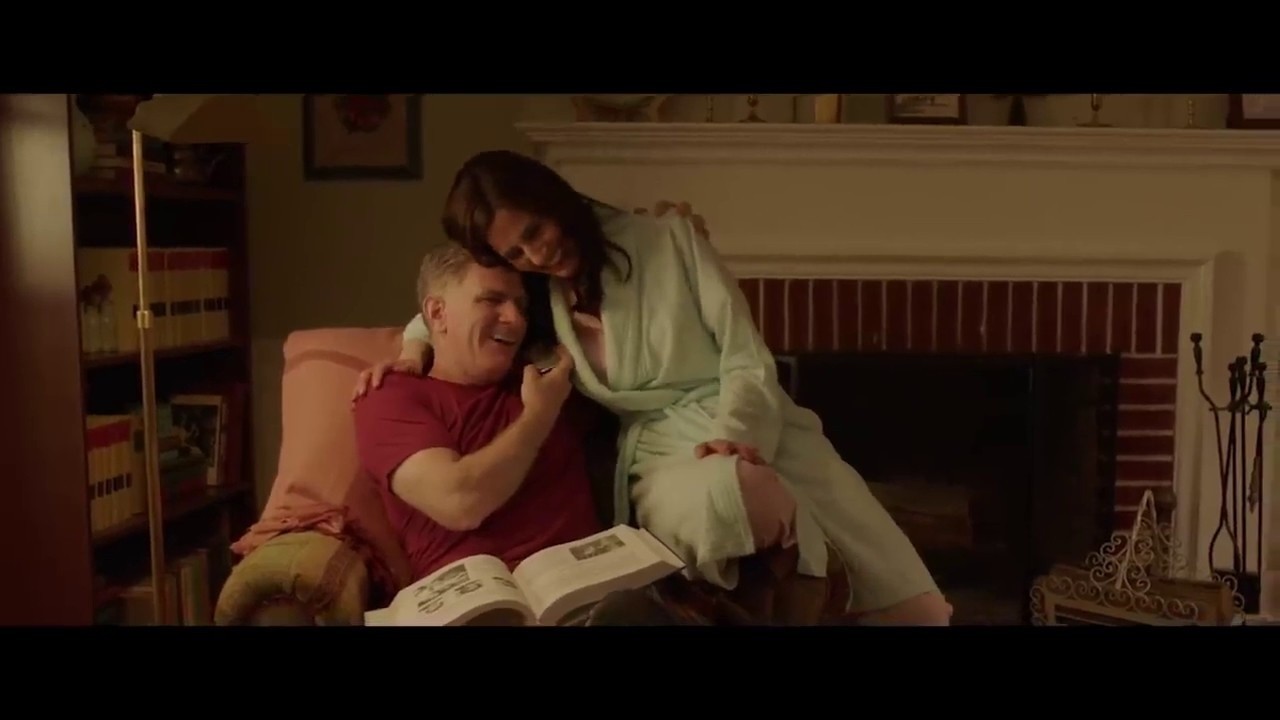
left=64, top=127, right=249, bottom=626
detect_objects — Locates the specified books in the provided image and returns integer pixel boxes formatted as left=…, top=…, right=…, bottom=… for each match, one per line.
left=364, top=524, right=687, bottom=627
left=79, top=246, right=248, bottom=625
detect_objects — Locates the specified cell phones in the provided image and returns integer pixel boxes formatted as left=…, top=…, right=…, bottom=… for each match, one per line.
left=522, top=324, right=562, bottom=375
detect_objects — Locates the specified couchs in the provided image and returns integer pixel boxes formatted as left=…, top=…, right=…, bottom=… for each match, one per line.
left=216, top=321, right=413, bottom=628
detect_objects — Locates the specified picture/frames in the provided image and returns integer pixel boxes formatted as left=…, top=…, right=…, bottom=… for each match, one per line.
left=883, top=94, right=967, bottom=125
left=300, top=92, right=423, bottom=180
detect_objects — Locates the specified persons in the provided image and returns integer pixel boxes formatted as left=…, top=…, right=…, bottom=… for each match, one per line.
left=355, top=201, right=709, bottom=626
left=350, top=149, right=954, bottom=626
left=323, top=94, right=407, bottom=168
